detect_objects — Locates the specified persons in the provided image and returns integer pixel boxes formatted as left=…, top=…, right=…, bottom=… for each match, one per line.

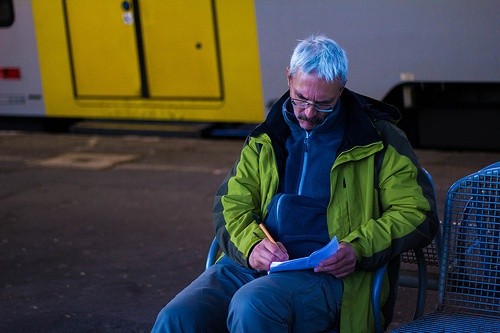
left=148, top=32, right=441, bottom=333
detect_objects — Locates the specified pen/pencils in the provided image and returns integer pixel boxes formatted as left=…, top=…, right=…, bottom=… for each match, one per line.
left=252, top=213, right=282, bottom=251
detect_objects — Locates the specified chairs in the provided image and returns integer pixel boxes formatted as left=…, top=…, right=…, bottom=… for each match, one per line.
left=205, top=161, right=500, bottom=333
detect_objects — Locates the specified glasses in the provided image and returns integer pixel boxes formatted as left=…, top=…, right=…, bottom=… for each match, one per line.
left=289, top=97, right=335, bottom=113
